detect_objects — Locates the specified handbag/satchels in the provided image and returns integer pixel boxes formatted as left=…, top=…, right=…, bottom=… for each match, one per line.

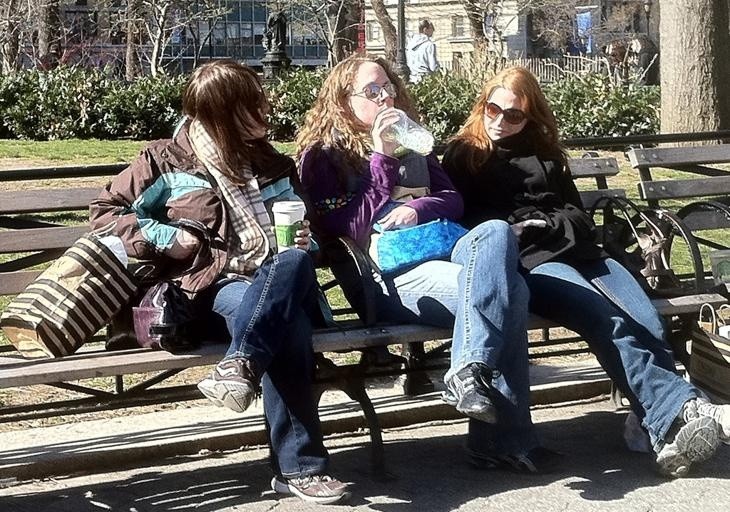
left=369, top=218, right=469, bottom=275
left=591, top=196, right=686, bottom=299
left=0, top=220, right=156, bottom=362
left=133, top=279, right=204, bottom=352
left=690, top=303, right=730, bottom=404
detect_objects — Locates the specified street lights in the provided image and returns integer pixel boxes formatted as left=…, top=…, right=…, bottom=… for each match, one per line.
left=644, top=0, right=652, bottom=38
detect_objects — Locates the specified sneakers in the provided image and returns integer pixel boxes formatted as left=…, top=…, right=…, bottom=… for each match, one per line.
left=648, top=397, right=730, bottom=479
left=440, top=362, right=499, bottom=424
left=467, top=433, right=567, bottom=475
left=270, top=470, right=352, bottom=505
left=196, top=357, right=258, bottom=412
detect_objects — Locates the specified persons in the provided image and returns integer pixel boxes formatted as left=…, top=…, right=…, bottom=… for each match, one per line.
left=300, top=49, right=565, bottom=474
left=90, top=59, right=360, bottom=504
left=440, top=66, right=730, bottom=478
left=404, top=19, right=443, bottom=84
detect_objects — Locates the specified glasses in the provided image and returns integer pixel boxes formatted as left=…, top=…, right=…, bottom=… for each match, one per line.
left=483, top=101, right=528, bottom=125
left=346, top=84, right=398, bottom=99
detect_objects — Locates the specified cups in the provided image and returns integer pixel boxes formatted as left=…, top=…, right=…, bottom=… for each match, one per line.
left=272, top=201, right=305, bottom=254
left=379, top=108, right=435, bottom=157
